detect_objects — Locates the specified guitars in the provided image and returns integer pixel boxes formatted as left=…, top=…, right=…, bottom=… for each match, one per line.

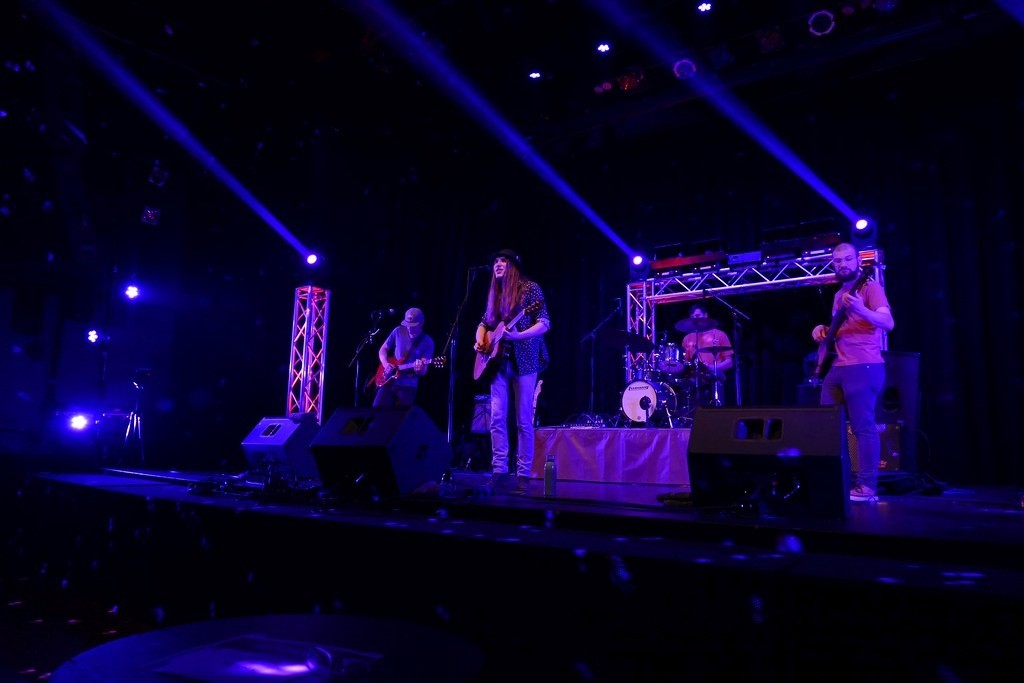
left=814, top=265, right=875, bottom=379
left=375, top=355, right=448, bottom=388
left=473, top=298, right=544, bottom=383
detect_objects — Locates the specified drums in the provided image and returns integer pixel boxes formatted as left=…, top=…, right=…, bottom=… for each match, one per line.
left=633, top=360, right=661, bottom=381
left=657, top=343, right=685, bottom=374
left=619, top=378, right=677, bottom=424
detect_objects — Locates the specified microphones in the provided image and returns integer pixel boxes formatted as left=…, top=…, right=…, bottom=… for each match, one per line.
left=374, top=309, right=395, bottom=315
left=619, top=299, right=624, bottom=316
left=702, top=288, right=706, bottom=303
left=470, top=265, right=491, bottom=272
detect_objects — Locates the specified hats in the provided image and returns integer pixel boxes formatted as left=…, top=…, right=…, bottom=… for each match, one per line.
left=492, top=249, right=524, bottom=273
left=401, top=308, right=425, bottom=327
left=690, top=304, right=708, bottom=318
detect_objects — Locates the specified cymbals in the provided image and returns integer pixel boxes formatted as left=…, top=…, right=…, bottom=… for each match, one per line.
left=699, top=345, right=734, bottom=353
left=605, top=330, right=655, bottom=353
left=674, top=318, right=719, bottom=333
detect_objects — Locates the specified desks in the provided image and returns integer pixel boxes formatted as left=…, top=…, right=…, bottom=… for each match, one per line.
left=530, top=426, right=690, bottom=488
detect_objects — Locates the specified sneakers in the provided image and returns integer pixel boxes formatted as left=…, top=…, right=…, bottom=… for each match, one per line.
left=850, top=485, right=879, bottom=501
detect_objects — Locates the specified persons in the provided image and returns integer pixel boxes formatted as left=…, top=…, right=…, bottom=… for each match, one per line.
left=683, top=303, right=734, bottom=371
left=372, top=307, right=435, bottom=406
left=812, top=243, right=894, bottom=502
left=474, top=248, right=552, bottom=476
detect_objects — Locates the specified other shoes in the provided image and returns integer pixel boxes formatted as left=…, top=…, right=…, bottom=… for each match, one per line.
left=511, top=476, right=530, bottom=495
left=483, top=473, right=508, bottom=488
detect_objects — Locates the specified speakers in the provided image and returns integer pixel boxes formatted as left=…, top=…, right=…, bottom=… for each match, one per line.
left=240, top=416, right=321, bottom=483
left=688, top=405, right=853, bottom=524
left=883, top=351, right=921, bottom=486
left=309, top=404, right=452, bottom=497
